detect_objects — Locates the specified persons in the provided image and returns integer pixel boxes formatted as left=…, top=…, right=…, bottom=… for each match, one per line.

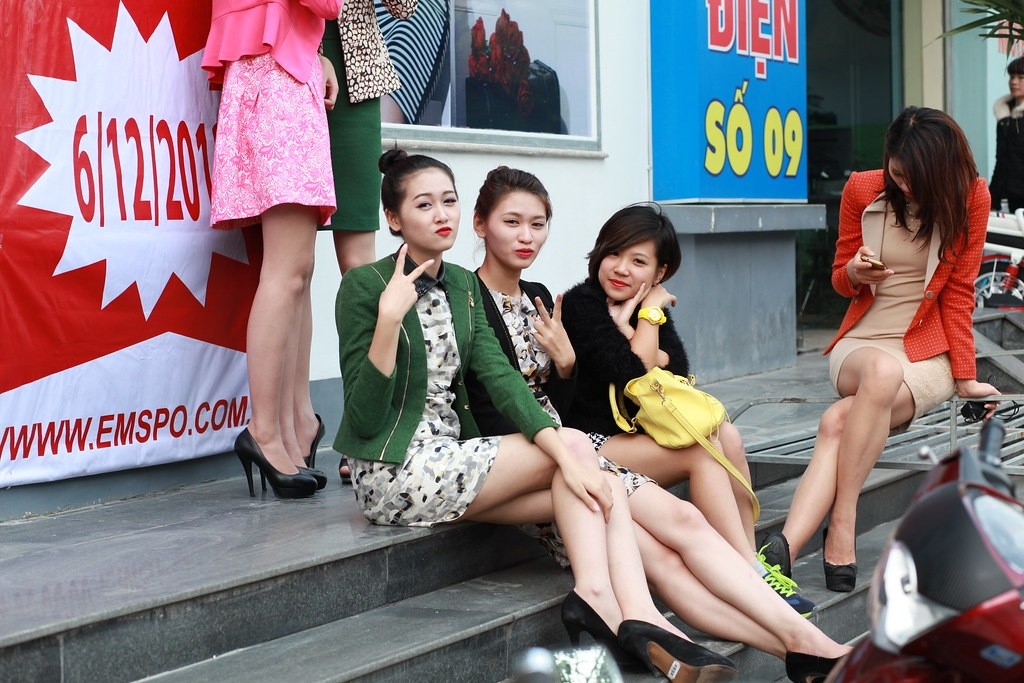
left=334, top=148, right=738, bottom=683
left=989, top=55, right=1024, bottom=213
left=761, top=107, right=1003, bottom=593
left=202, top=0, right=417, bottom=501
left=461, top=166, right=853, bottom=683
left=551, top=204, right=817, bottom=618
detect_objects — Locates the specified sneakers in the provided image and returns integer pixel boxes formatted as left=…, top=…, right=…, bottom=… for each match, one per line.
left=755, top=540, right=817, bottom=619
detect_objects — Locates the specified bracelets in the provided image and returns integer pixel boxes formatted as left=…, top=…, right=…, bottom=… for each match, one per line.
left=629, top=332, right=635, bottom=341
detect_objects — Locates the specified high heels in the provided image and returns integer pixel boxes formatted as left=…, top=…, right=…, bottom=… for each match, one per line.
left=760, top=532, right=792, bottom=579
left=234, top=426, right=318, bottom=499
left=823, top=526, right=857, bottom=592
left=561, top=586, right=650, bottom=673
left=337, top=457, right=362, bottom=484
left=302, top=412, right=325, bottom=468
left=616, top=619, right=738, bottom=683
left=259, top=441, right=327, bottom=491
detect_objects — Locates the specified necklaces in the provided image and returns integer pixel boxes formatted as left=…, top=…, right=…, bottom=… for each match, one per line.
left=907, top=201, right=921, bottom=231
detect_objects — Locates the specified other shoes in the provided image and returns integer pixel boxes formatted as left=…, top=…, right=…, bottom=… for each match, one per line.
left=785, top=650, right=851, bottom=683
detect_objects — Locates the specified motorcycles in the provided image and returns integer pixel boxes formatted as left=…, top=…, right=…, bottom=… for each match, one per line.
left=975, top=209, right=1024, bottom=311
left=823, top=418, right=1024, bottom=683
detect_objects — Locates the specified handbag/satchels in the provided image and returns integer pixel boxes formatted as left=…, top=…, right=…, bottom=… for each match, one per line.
left=609, top=371, right=725, bottom=448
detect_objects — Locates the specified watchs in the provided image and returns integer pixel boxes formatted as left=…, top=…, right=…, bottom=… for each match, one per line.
left=637, top=308, right=667, bottom=326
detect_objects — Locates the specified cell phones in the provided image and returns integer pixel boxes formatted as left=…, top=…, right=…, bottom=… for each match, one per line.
left=860, top=255, right=887, bottom=271
